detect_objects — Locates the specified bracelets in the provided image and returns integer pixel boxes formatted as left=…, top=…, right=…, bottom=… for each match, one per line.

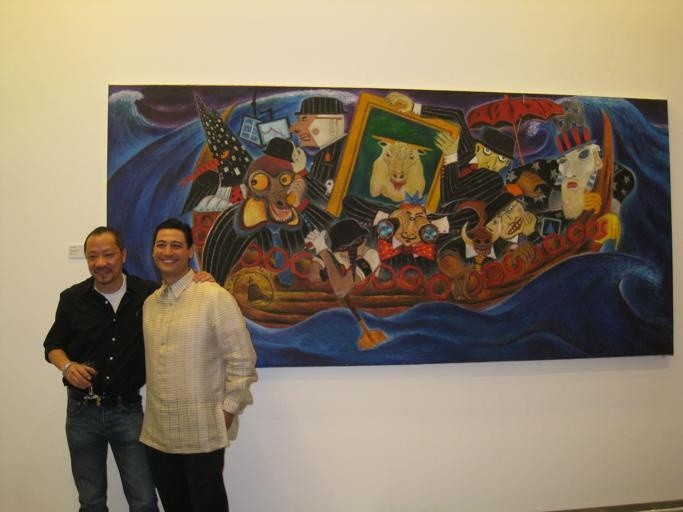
left=63, top=361, right=76, bottom=379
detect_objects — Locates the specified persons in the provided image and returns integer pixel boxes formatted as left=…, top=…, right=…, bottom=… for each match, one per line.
left=139, top=219, right=258, bottom=512
left=43, top=226, right=217, bottom=511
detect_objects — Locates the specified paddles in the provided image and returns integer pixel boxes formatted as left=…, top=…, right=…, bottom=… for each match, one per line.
left=305, top=243, right=388, bottom=352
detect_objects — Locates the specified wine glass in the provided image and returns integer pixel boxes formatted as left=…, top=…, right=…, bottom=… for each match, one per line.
left=83, top=360, right=99, bottom=401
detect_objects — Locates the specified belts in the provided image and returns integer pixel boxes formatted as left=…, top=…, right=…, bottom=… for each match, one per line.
left=67, top=393, right=142, bottom=408
